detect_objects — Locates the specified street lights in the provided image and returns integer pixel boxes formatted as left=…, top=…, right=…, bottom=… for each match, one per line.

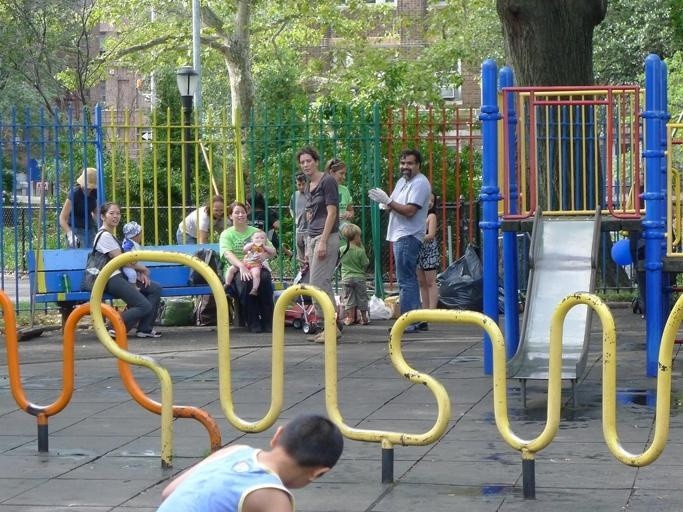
left=174, top=66, right=198, bottom=218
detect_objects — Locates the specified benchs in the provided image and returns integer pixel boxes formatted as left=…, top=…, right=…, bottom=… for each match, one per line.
left=27, top=244, right=289, bottom=326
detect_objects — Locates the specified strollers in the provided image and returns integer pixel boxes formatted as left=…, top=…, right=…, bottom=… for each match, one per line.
left=271, top=235, right=349, bottom=333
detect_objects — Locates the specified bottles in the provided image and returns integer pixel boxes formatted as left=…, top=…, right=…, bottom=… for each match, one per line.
left=62, top=273, right=71, bottom=294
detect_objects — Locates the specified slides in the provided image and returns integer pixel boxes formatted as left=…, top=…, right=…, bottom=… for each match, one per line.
left=504, top=206, right=602, bottom=410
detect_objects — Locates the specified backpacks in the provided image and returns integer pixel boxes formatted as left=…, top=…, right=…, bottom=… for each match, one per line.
left=188, top=248, right=224, bottom=287
left=195, top=295, right=234, bottom=326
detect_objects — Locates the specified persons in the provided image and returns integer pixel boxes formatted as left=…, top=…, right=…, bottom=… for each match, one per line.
left=176, top=148, right=371, bottom=343
left=156, top=414, right=344, bottom=512
left=92, top=202, right=163, bottom=338
left=121, top=220, right=144, bottom=291
left=58, top=167, right=100, bottom=249
left=415, top=191, right=440, bottom=310
left=367, top=149, right=431, bottom=334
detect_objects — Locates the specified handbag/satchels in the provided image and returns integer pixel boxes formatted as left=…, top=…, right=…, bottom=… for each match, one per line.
left=80, top=230, right=111, bottom=293
left=159, top=299, right=194, bottom=326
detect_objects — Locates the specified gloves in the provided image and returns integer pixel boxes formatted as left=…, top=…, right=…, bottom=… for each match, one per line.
left=67, top=232, right=80, bottom=249
left=368, top=188, right=392, bottom=206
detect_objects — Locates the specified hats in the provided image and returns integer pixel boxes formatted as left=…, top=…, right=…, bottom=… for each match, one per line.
left=122, top=221, right=142, bottom=240
left=77, top=168, right=97, bottom=190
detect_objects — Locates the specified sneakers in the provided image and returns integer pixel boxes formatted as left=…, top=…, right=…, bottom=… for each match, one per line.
left=359, top=319, right=368, bottom=325
left=388, top=325, right=416, bottom=333
left=135, top=328, right=162, bottom=338
left=414, top=321, right=428, bottom=331
left=315, top=328, right=341, bottom=343
left=105, top=324, right=116, bottom=339
left=306, top=331, right=324, bottom=341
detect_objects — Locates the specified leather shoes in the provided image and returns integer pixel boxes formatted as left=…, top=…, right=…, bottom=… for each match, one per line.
left=247, top=321, right=261, bottom=333
left=262, top=321, right=271, bottom=333
